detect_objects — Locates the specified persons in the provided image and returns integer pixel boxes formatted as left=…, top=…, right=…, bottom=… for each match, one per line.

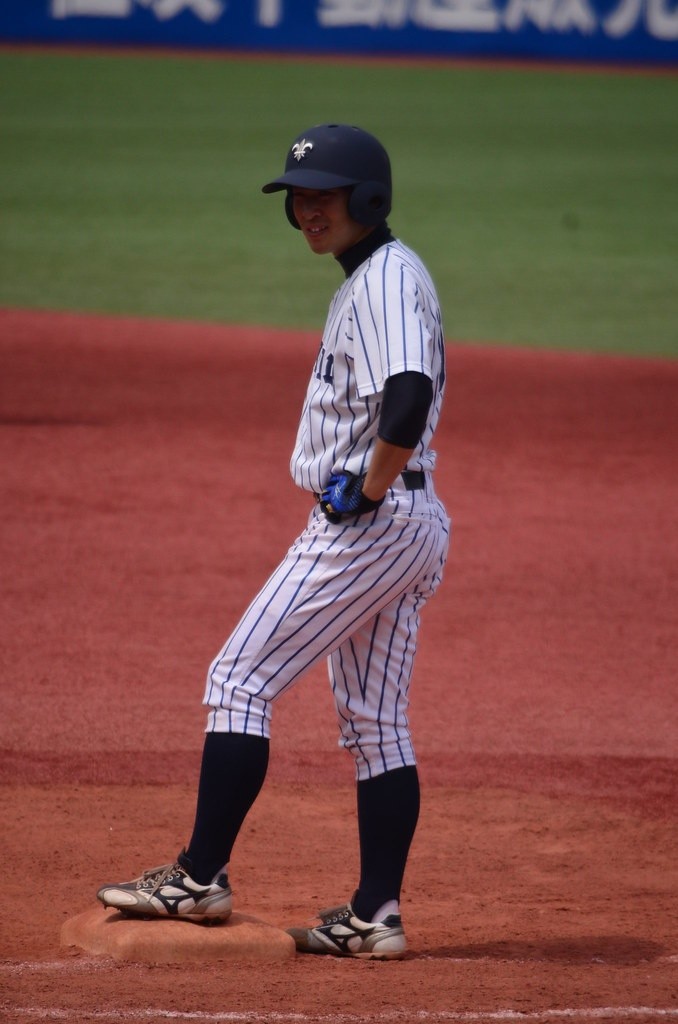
left=94, top=125, right=452, bottom=960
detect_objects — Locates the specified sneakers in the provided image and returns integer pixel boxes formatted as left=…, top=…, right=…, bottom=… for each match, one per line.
left=96, top=845, right=232, bottom=923
left=284, top=889, right=407, bottom=960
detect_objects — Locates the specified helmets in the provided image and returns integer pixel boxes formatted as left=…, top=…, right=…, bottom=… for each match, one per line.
left=261, top=124, right=391, bottom=230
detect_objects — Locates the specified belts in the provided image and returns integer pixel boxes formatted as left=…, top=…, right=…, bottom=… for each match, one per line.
left=401, top=472, right=426, bottom=489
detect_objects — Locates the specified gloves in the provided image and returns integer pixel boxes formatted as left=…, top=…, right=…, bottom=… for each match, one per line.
left=316, top=470, right=386, bottom=524
